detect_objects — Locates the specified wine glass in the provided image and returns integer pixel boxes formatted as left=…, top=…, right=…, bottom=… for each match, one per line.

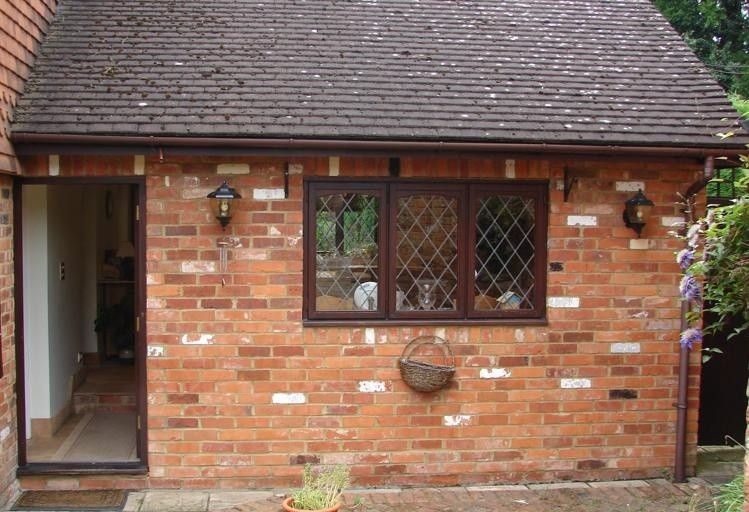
left=417, top=279, right=437, bottom=311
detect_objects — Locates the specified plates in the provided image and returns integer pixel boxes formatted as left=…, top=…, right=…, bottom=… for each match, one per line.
left=354, top=282, right=378, bottom=311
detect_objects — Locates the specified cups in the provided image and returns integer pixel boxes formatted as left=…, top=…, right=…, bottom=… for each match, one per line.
left=396, top=290, right=404, bottom=310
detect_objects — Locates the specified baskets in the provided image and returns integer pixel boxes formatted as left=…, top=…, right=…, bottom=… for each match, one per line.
left=398, top=335, right=455, bottom=392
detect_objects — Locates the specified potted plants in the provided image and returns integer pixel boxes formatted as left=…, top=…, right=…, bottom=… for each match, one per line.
left=282, top=463, right=352, bottom=512
left=96, top=285, right=136, bottom=364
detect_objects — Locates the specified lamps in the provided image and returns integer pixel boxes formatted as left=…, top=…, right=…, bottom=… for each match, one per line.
left=115, top=239, right=135, bottom=280
left=623, top=188, right=655, bottom=238
left=207, top=180, right=242, bottom=230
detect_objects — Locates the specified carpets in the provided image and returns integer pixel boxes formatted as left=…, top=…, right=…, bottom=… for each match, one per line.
left=10, top=489, right=128, bottom=512
